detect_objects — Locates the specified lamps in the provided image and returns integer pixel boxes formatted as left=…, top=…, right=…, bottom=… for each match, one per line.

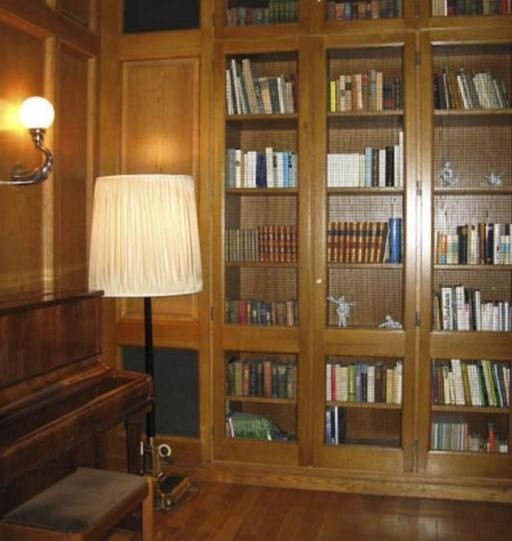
left=0, top=96, right=55, bottom=186
left=88, top=173, right=205, bottom=510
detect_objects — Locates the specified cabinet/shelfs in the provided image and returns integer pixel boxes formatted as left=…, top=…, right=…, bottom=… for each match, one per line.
left=207, top=1, right=417, bottom=499
left=417, top=0, right=512, bottom=504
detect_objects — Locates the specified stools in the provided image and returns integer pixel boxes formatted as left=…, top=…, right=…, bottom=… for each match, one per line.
left=1, top=468, right=156, bottom=541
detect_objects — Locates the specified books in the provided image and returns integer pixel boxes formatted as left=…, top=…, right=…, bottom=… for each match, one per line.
left=324, top=407, right=344, bottom=447
left=222, top=359, right=298, bottom=401
left=222, top=0, right=299, bottom=28
left=428, top=0, right=512, bottom=18
left=326, top=131, right=406, bottom=189
left=324, top=358, right=404, bottom=407
left=432, top=285, right=512, bottom=333
left=225, top=300, right=300, bottom=329
left=327, top=217, right=403, bottom=263
left=432, top=66, right=512, bottom=110
left=224, top=57, right=297, bottom=114
left=430, top=416, right=510, bottom=455
left=434, top=222, right=512, bottom=267
left=431, top=357, right=512, bottom=410
left=327, top=67, right=400, bottom=112
left=225, top=140, right=298, bottom=188
left=326, top=0, right=406, bottom=20
left=223, top=223, right=298, bottom=262
